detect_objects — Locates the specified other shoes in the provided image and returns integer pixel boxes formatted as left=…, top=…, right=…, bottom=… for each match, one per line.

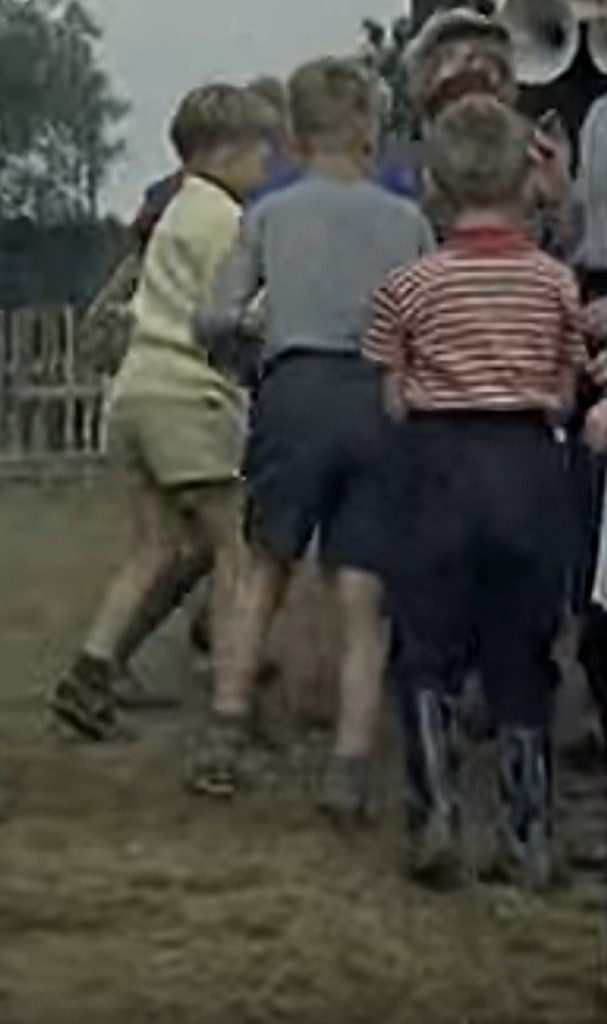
left=184, top=761, right=238, bottom=799
left=42, top=648, right=125, bottom=743
left=406, top=827, right=468, bottom=891
left=477, top=836, right=570, bottom=889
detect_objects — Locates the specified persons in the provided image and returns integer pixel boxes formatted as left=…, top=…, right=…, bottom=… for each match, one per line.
left=44, top=9, right=607, bottom=884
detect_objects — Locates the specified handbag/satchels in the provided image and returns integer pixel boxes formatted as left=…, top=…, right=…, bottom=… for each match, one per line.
left=75, top=251, right=143, bottom=374
